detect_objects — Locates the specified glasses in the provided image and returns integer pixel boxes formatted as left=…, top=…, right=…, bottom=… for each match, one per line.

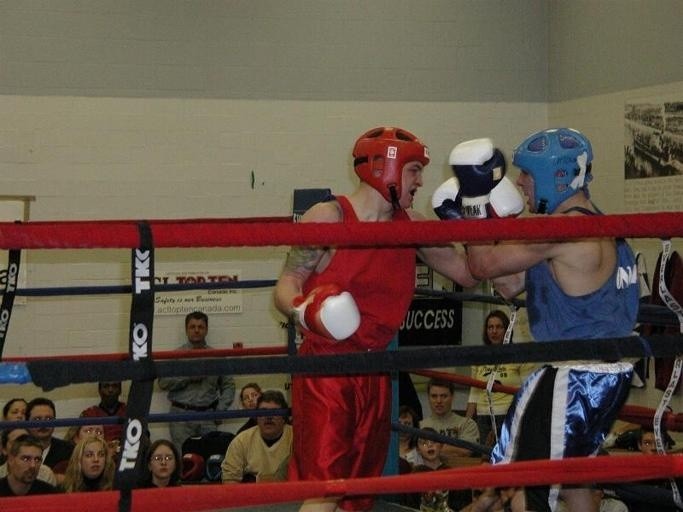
left=84, top=429, right=105, bottom=436
left=151, top=456, right=175, bottom=462
left=418, top=440, right=435, bottom=446
left=243, top=391, right=258, bottom=402
left=16, top=456, right=43, bottom=462
left=643, top=440, right=656, bottom=447
left=32, top=417, right=54, bottom=420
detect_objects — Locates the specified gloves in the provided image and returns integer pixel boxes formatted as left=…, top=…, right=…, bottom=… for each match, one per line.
left=431, top=177, right=461, bottom=220
left=292, top=285, right=361, bottom=340
left=450, top=138, right=507, bottom=218
left=487, top=177, right=525, bottom=219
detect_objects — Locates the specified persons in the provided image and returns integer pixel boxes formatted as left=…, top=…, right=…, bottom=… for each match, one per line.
left=0, top=398, right=27, bottom=421
left=54, top=425, right=104, bottom=475
left=67, top=381, right=127, bottom=440
left=55, top=433, right=116, bottom=494
left=0, top=428, right=57, bottom=487
left=119, top=440, right=182, bottom=512
left=418, top=379, right=480, bottom=457
left=1, top=434, right=64, bottom=498
left=399, top=406, right=420, bottom=464
left=465, top=310, right=535, bottom=444
left=221, top=391, right=293, bottom=483
left=158, top=311, right=235, bottom=461
left=237, top=383, right=263, bottom=436
left=402, top=427, right=473, bottom=512
left=636, top=424, right=667, bottom=454
left=274, top=126, right=524, bottom=512
left=432, top=128, right=640, bottom=512
left=0, top=398, right=75, bottom=470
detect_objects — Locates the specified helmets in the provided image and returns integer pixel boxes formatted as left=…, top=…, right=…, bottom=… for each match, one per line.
left=180, top=453, right=204, bottom=482
left=205, top=454, right=225, bottom=481
left=353, top=128, right=431, bottom=214
left=511, top=128, right=593, bottom=213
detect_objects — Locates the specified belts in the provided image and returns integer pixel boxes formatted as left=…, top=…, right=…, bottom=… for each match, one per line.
left=171, top=400, right=220, bottom=412
